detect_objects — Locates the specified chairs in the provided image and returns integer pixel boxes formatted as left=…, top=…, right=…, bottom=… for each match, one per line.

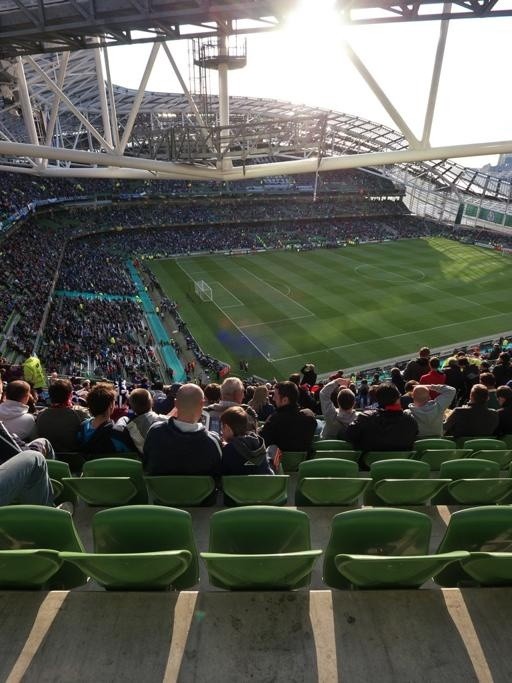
left=414, top=440, right=469, bottom=470
left=61, top=457, right=144, bottom=507
left=368, top=458, right=453, bottom=506
left=433, top=505, right=512, bottom=586
left=142, top=475, right=214, bottom=505
left=199, top=505, right=323, bottom=591
left=41, top=457, right=77, bottom=504
left=0, top=505, right=90, bottom=591
left=462, top=439, right=512, bottom=468
left=58, top=504, right=199, bottom=593
left=223, top=475, right=288, bottom=505
left=364, top=451, right=417, bottom=469
left=323, top=507, right=469, bottom=590
left=297, top=458, right=373, bottom=506
left=440, top=457, right=512, bottom=504
left=313, top=441, right=363, bottom=463
left=279, top=450, right=308, bottom=471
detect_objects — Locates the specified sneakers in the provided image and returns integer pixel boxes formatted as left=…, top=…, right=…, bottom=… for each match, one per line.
left=57, top=500, right=74, bottom=516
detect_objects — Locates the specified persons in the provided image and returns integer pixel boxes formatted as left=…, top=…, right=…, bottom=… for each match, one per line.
left=350, top=373, right=356, bottom=384
left=47, top=373, right=59, bottom=386
left=496, top=386, right=512, bottom=435
left=301, top=364, right=316, bottom=386
left=369, top=375, right=381, bottom=405
left=127, top=387, right=170, bottom=452
left=23, top=345, right=46, bottom=401
left=259, top=381, right=317, bottom=453
left=289, top=373, right=320, bottom=407
left=391, top=368, right=404, bottom=392
left=319, top=376, right=363, bottom=441
left=471, top=353, right=481, bottom=366
left=244, top=386, right=255, bottom=404
left=330, top=371, right=343, bottom=387
left=458, top=357, right=477, bottom=374
left=219, top=406, right=282, bottom=477
left=420, top=357, right=446, bottom=398
left=441, top=351, right=465, bottom=368
left=365, top=385, right=379, bottom=410
left=36, top=377, right=81, bottom=449
left=444, top=384, right=499, bottom=437
left=0, top=419, right=55, bottom=462
left=169, top=337, right=183, bottom=354
left=201, top=376, right=245, bottom=435
left=79, top=381, right=96, bottom=399
left=492, top=352, right=512, bottom=385
left=0, top=448, right=74, bottom=515
left=185, top=359, right=195, bottom=375
left=479, top=373, right=500, bottom=410
left=149, top=382, right=166, bottom=411
left=239, top=360, right=249, bottom=373
left=204, top=383, right=221, bottom=404
left=361, top=380, right=368, bottom=404
left=77, top=382, right=137, bottom=459
left=489, top=344, right=500, bottom=359
left=346, top=382, right=419, bottom=451
left=247, top=386, right=275, bottom=421
left=142, top=383, right=222, bottom=481
left=0, top=380, right=35, bottom=441
left=76, top=379, right=90, bottom=395
left=403, top=347, right=431, bottom=380
left=444, top=358, right=459, bottom=374
left=471, top=345, right=480, bottom=352
left=140, top=378, right=150, bottom=390
left=160, top=383, right=182, bottom=413
left=404, top=384, right=457, bottom=437
left=480, top=362, right=490, bottom=374
left=156, top=301, right=174, bottom=319
left=401, top=379, right=420, bottom=410
